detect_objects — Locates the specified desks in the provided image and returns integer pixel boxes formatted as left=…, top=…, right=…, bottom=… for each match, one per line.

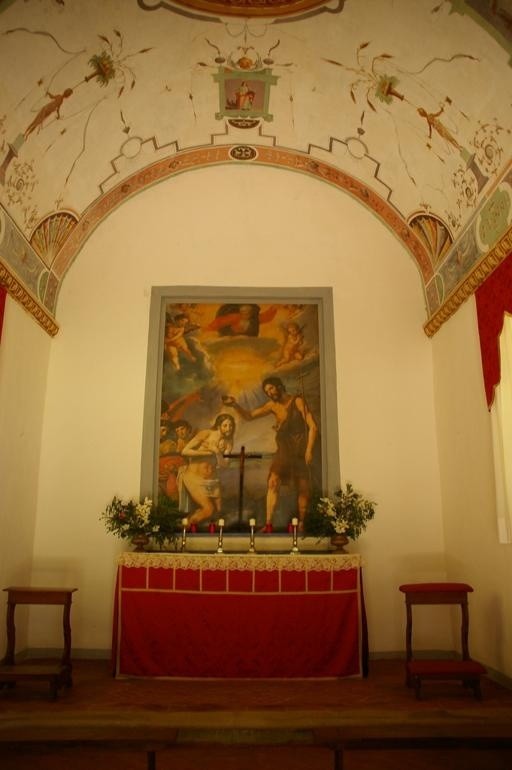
left=111, top=551, right=369, bottom=681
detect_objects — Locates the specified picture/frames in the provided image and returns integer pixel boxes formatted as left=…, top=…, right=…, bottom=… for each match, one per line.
left=139, top=286, right=341, bottom=552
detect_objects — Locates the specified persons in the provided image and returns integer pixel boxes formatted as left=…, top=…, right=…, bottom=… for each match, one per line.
left=164, top=313, right=197, bottom=371
left=274, top=323, right=304, bottom=368
left=222, top=377, right=318, bottom=533
left=203, top=304, right=279, bottom=337
left=159, top=413, right=235, bottom=530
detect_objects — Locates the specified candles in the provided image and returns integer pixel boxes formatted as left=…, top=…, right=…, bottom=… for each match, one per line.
left=292, top=518, right=298, bottom=525
left=250, top=519, right=255, bottom=525
left=183, top=519, right=187, bottom=525
left=219, top=519, right=224, bottom=525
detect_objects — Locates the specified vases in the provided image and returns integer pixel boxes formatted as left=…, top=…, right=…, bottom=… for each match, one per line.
left=132, top=533, right=150, bottom=552
left=331, top=535, right=349, bottom=553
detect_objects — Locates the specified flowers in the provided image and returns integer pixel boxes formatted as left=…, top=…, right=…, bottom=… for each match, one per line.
left=100, top=491, right=189, bottom=548
left=304, top=482, right=377, bottom=544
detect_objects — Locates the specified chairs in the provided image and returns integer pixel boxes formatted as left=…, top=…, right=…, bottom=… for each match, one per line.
left=398, top=583, right=487, bottom=700
left=0, top=586, right=78, bottom=699
